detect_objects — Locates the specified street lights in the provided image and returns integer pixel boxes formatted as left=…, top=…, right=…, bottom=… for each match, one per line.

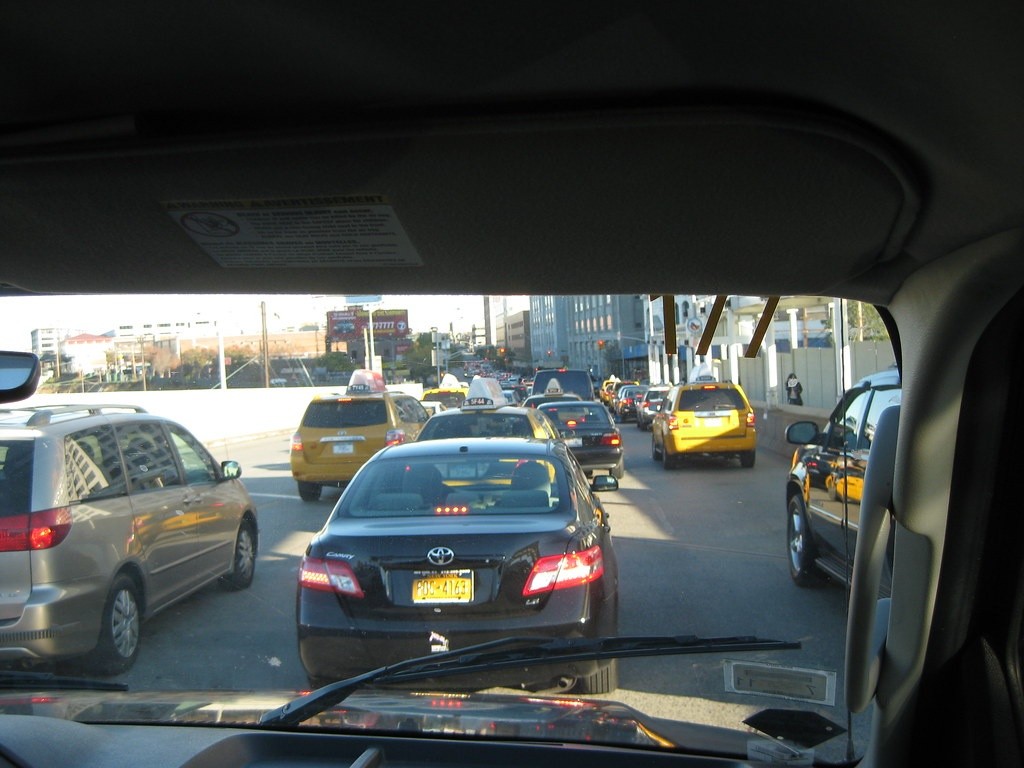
left=430, top=326, right=441, bottom=388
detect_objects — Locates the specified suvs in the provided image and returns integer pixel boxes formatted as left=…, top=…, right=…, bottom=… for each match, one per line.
left=290, top=369, right=430, bottom=503
left=650, top=361, right=759, bottom=472
left=782, top=367, right=903, bottom=591
left=0, top=402, right=261, bottom=677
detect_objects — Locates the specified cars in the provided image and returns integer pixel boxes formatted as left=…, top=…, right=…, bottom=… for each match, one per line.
left=533, top=400, right=627, bottom=482
left=294, top=435, right=619, bottom=703
left=403, top=377, right=562, bottom=495
left=418, top=401, right=449, bottom=419
left=461, top=359, right=674, bottom=433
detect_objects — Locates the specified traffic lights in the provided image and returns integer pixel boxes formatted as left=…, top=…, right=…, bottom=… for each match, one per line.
left=500, top=347, right=506, bottom=357
left=547, top=351, right=551, bottom=357
left=598, top=339, right=605, bottom=352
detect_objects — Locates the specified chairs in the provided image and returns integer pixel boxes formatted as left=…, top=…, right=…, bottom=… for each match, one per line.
left=585, top=415, right=600, bottom=422
left=511, top=461, right=550, bottom=497
left=78, top=441, right=111, bottom=485
left=550, top=411, right=559, bottom=425
left=402, top=463, right=442, bottom=493
left=512, top=421, right=528, bottom=435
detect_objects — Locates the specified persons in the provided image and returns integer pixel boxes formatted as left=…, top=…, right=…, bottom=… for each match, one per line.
left=786, top=374, right=803, bottom=407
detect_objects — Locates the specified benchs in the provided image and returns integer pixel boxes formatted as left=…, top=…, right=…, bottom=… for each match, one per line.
left=372, top=490, right=551, bottom=511
